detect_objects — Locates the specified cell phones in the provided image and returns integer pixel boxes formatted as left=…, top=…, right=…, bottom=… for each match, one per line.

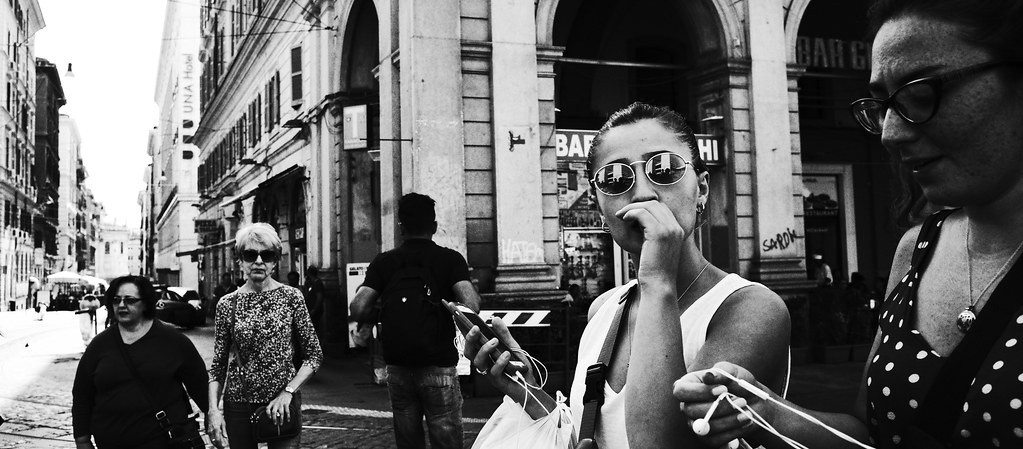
left=448, top=301, right=529, bottom=376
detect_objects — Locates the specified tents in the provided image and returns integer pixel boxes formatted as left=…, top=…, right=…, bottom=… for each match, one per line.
left=46, top=271, right=110, bottom=294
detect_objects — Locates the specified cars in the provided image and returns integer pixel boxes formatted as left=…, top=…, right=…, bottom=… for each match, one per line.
left=166, top=286, right=207, bottom=327
left=154, top=288, right=200, bottom=331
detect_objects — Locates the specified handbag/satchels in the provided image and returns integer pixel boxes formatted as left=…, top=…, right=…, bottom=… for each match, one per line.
left=472, top=391, right=573, bottom=449
left=251, top=406, right=297, bottom=443
left=170, top=412, right=206, bottom=449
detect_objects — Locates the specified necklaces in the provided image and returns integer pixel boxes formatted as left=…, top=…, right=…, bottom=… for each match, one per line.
left=954, top=212, right=1023, bottom=333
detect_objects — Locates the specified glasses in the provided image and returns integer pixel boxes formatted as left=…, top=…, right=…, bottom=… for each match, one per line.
left=241, top=248, right=276, bottom=262
left=850, top=59, right=1008, bottom=137
left=112, top=295, right=141, bottom=305
left=588, top=153, right=700, bottom=198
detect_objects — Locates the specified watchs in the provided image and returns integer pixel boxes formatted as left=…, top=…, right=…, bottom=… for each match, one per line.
left=285, top=386, right=296, bottom=398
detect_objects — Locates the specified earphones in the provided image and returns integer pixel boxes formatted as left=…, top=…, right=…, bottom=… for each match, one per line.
left=693, top=400, right=719, bottom=436
left=723, top=438, right=740, bottom=449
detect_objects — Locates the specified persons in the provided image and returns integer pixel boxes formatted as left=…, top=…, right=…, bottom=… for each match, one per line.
left=349, top=191, right=483, bottom=449
left=88, top=296, right=99, bottom=335
left=101, top=285, right=105, bottom=294
left=71, top=274, right=210, bottom=449
left=83, top=286, right=88, bottom=294
left=671, top=0, right=1023, bottom=449
left=447, top=102, right=792, bottom=449
left=213, top=273, right=237, bottom=316
left=271, top=264, right=324, bottom=370
left=205, top=221, right=325, bottom=449
left=34, top=289, right=47, bottom=321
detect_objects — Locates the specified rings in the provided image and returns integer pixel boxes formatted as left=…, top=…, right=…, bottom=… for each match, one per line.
left=475, top=367, right=489, bottom=376
